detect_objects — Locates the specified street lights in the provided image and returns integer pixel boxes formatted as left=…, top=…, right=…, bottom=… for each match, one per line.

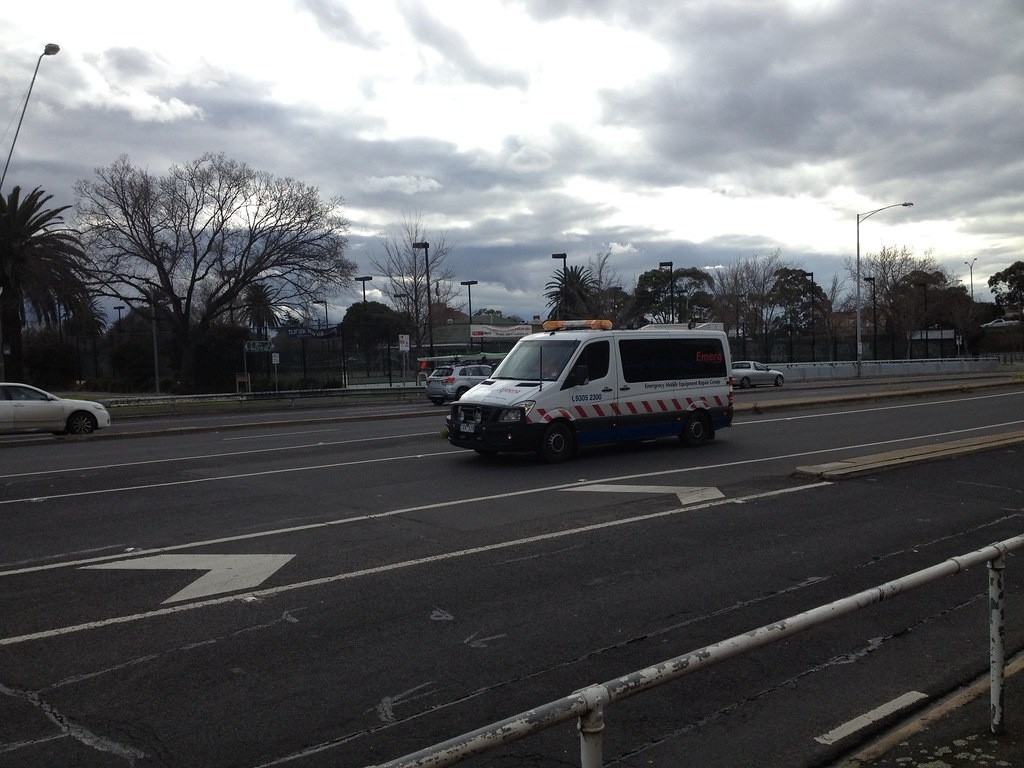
left=313, top=299, right=332, bottom=352
left=460, top=278, right=478, bottom=356
left=112, top=305, right=128, bottom=335
left=800, top=271, right=816, bottom=363
left=917, top=283, right=928, bottom=360
left=551, top=253, right=571, bottom=319
left=659, top=262, right=676, bottom=324
left=855, top=201, right=914, bottom=378
left=414, top=242, right=438, bottom=359
left=863, top=275, right=878, bottom=361
left=963, top=257, right=978, bottom=300
left=1, top=42, right=59, bottom=198
left=353, top=275, right=377, bottom=378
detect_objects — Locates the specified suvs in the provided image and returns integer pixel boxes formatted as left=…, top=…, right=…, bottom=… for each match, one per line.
left=423, top=363, right=495, bottom=406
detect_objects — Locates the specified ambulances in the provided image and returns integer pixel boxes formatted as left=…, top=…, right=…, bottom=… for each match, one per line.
left=445, top=318, right=735, bottom=461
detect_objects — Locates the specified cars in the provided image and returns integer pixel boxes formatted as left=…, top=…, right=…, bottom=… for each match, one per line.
left=980, top=318, right=1020, bottom=328
left=0, top=382, right=113, bottom=438
left=729, top=359, right=786, bottom=389
